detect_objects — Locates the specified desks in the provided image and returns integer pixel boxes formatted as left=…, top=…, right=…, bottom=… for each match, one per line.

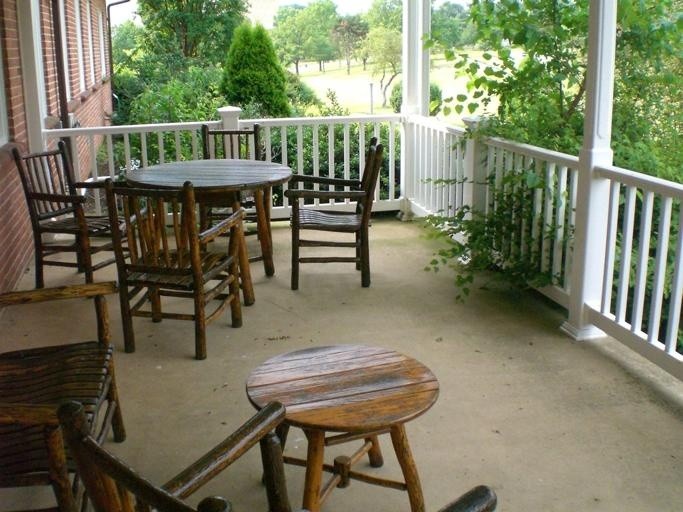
left=241, top=341, right=444, bottom=509
left=120, top=156, right=296, bottom=284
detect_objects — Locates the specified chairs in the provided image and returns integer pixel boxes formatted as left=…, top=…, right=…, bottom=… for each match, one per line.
left=10, top=142, right=130, bottom=293
left=282, top=136, right=386, bottom=291
left=198, top=123, right=276, bottom=250
left=103, top=176, right=247, bottom=362
left=1, top=276, right=127, bottom=510
left=57, top=396, right=498, bottom=510
